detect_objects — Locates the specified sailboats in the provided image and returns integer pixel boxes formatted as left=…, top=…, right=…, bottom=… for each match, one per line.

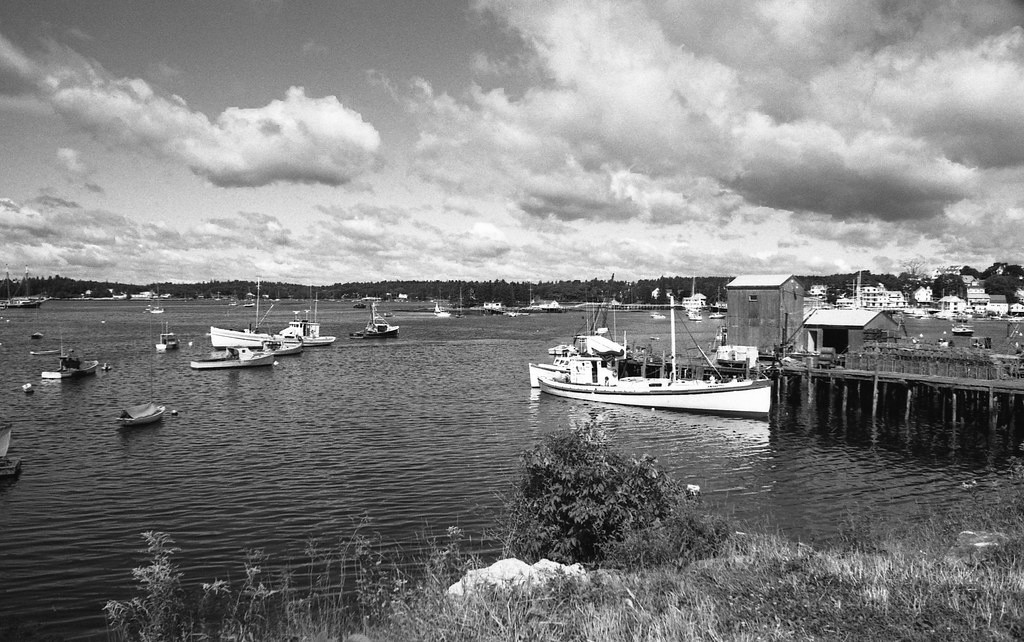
left=685, top=282, right=702, bottom=321
left=707, top=287, right=727, bottom=319
left=0, top=265, right=49, bottom=310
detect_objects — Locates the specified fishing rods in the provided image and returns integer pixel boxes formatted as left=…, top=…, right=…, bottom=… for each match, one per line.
left=54, top=312, right=63, bottom=356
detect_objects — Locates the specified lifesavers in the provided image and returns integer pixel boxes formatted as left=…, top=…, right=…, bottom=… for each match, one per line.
left=298, top=336, right=302, bottom=341
left=612, top=370, right=618, bottom=377
left=636, top=342, right=652, bottom=356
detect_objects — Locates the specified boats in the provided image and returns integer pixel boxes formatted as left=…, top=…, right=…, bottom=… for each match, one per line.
left=115, top=361, right=166, bottom=426
left=154, top=321, right=180, bottom=352
left=951, top=316, right=974, bottom=336
left=189, top=278, right=337, bottom=370
left=0, top=424, right=23, bottom=477
left=529, top=271, right=774, bottom=419
left=650, top=308, right=667, bottom=320
left=348, top=300, right=401, bottom=340
left=39, top=333, right=100, bottom=379
left=432, top=284, right=567, bottom=318
